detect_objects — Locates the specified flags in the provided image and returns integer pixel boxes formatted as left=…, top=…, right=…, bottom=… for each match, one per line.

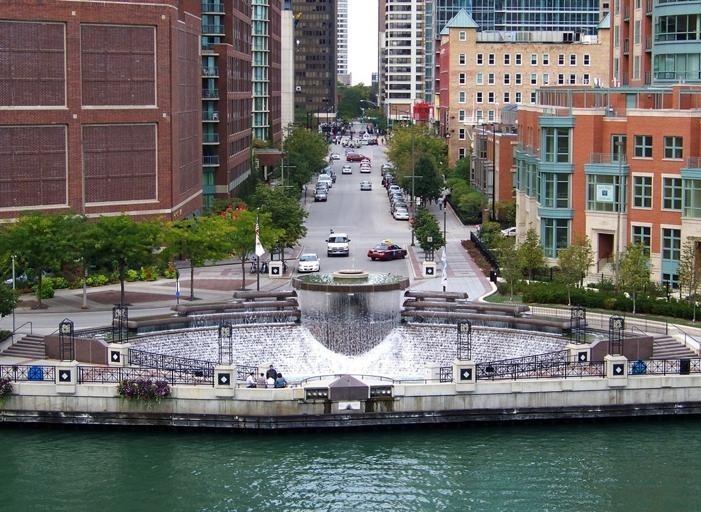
left=255, top=216, right=266, bottom=257
left=441, top=252, right=448, bottom=287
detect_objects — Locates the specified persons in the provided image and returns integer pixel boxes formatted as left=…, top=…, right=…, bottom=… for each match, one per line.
left=332, top=172, right=336, bottom=183
left=330, top=229, right=334, bottom=234
left=245, top=365, right=288, bottom=388
left=437, top=196, right=444, bottom=210
left=382, top=138, right=383, bottom=144
left=428, top=197, right=432, bottom=205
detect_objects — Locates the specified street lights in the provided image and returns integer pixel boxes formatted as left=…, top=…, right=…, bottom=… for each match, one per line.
left=9, top=253, right=18, bottom=308
left=358, top=100, right=378, bottom=110
left=441, top=186, right=451, bottom=254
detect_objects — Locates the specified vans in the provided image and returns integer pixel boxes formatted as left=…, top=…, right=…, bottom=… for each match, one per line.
left=326, top=232, right=351, bottom=256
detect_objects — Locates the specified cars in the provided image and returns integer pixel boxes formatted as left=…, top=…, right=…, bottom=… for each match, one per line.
left=2, top=267, right=57, bottom=289
left=368, top=240, right=408, bottom=261
left=297, top=252, right=321, bottom=272
left=499, top=225, right=516, bottom=238
left=314, top=116, right=410, bottom=220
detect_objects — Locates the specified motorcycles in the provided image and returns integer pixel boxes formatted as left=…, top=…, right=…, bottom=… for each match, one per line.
left=250, top=258, right=288, bottom=274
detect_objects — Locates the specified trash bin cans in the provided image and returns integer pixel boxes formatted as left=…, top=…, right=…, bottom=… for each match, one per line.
left=680, top=359, right=690, bottom=375
left=490, top=271, right=497, bottom=284
